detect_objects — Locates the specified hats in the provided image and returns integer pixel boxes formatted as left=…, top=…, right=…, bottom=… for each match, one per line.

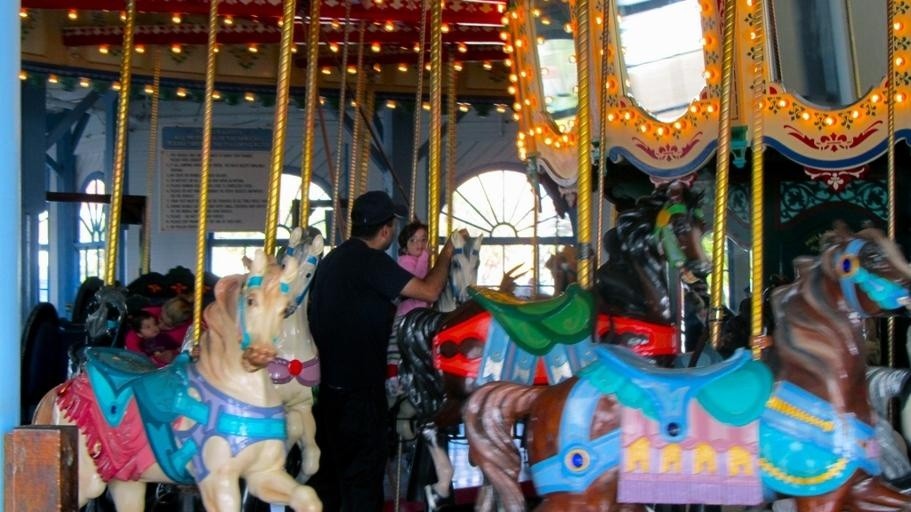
left=351, top=191, right=409, bottom=222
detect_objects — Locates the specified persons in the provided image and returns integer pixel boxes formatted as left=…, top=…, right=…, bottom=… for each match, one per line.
left=301, top=191, right=468, bottom=511
left=124, top=299, right=191, bottom=365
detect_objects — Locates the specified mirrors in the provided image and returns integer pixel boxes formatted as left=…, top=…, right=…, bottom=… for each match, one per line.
left=504, top=0, right=602, bottom=207
left=594, top=0, right=749, bottom=182
left=735, top=0, right=910, bottom=173
